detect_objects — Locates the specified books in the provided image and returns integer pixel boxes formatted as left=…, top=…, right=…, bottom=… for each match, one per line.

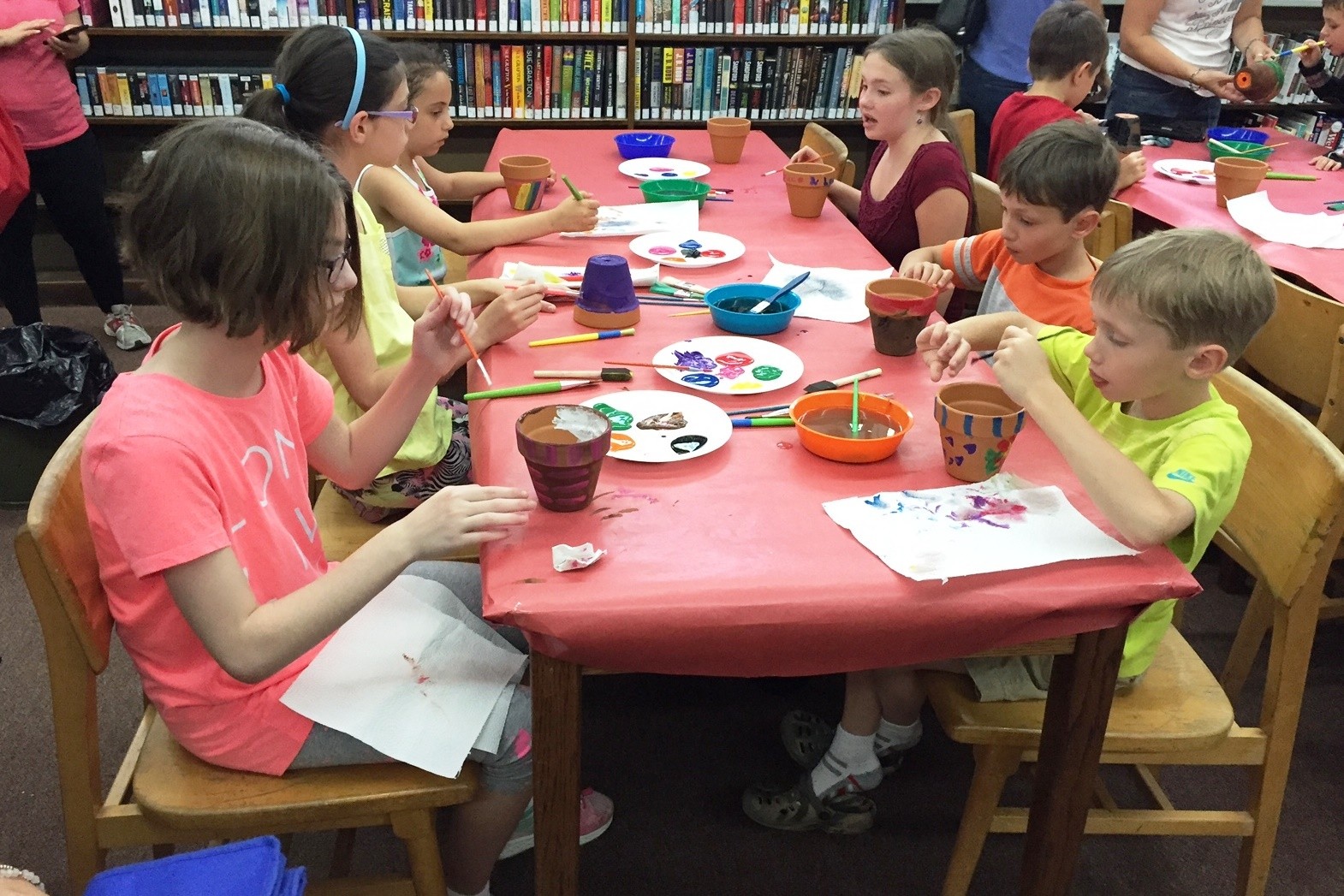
left=64, top=0, right=1344, bottom=157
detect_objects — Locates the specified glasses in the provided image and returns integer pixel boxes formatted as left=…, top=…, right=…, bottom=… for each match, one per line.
left=334, top=103, right=418, bottom=128
left=317, top=241, right=351, bottom=284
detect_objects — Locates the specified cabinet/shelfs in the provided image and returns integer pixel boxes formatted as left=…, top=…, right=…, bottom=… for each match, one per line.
left=20, top=1, right=1344, bottom=303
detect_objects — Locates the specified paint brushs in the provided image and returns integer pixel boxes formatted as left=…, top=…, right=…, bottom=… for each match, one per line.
left=1324, top=200, right=1344, bottom=204
left=529, top=328, right=636, bottom=346
left=627, top=186, right=734, bottom=202
left=667, top=311, right=711, bottom=317
left=731, top=418, right=794, bottom=425
left=760, top=151, right=836, bottom=177
left=604, top=359, right=713, bottom=372
left=1243, top=142, right=1289, bottom=154
left=561, top=175, right=582, bottom=200
left=970, top=332, right=1057, bottom=363
left=642, top=302, right=709, bottom=306
left=503, top=285, right=582, bottom=297
left=424, top=267, right=493, bottom=387
left=1266, top=173, right=1316, bottom=180
left=1209, top=138, right=1242, bottom=154
left=636, top=296, right=704, bottom=302
left=727, top=393, right=893, bottom=416
left=852, top=377, right=858, bottom=432
left=1270, top=41, right=1326, bottom=60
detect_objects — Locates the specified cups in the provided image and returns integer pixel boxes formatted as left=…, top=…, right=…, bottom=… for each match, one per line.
left=864, top=277, right=939, bottom=355
left=574, top=254, right=641, bottom=328
left=707, top=117, right=751, bottom=164
left=1213, top=156, right=1268, bottom=207
left=933, top=381, right=1029, bottom=482
left=1234, top=60, right=1285, bottom=102
left=517, top=404, right=612, bottom=513
left=499, top=155, right=551, bottom=210
left=783, top=163, right=836, bottom=217
left=1109, top=113, right=1142, bottom=154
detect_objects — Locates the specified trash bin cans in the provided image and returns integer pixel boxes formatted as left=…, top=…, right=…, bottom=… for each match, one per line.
left=0, top=323, right=97, bottom=508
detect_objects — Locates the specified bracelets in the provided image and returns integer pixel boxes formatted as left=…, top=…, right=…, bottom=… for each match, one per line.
left=1246, top=38, right=1265, bottom=56
left=1190, top=68, right=1205, bottom=91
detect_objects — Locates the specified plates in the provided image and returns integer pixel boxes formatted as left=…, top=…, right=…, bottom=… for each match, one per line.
left=577, top=391, right=734, bottom=463
left=653, top=336, right=804, bottom=394
left=1153, top=158, right=1217, bottom=185
left=629, top=230, right=747, bottom=268
left=618, top=157, right=712, bottom=182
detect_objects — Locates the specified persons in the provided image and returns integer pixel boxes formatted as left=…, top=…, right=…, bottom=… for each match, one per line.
left=0, top=1, right=619, bottom=896
left=744, top=0, right=1344, bottom=836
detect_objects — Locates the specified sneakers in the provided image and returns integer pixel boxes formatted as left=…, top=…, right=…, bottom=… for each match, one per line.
left=103, top=303, right=151, bottom=351
left=499, top=786, right=613, bottom=860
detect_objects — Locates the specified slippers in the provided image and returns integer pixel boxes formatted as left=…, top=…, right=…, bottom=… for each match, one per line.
left=776, top=708, right=904, bottom=773
left=743, top=773, right=875, bottom=837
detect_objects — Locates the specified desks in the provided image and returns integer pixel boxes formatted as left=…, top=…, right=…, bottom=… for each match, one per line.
left=1114, top=127, right=1344, bottom=301
left=470, top=128, right=1202, bottom=896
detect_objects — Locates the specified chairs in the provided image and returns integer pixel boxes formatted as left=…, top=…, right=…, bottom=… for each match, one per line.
left=800, top=109, right=1344, bottom=896
left=14, top=244, right=476, bottom=895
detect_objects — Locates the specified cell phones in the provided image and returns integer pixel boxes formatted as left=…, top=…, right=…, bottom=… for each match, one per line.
left=43, top=24, right=88, bottom=44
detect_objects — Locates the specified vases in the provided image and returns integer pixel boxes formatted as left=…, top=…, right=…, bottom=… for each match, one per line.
left=783, top=162, right=836, bottom=218
left=1214, top=157, right=1269, bottom=208
left=866, top=277, right=939, bottom=357
left=499, top=155, right=551, bottom=211
left=935, top=381, right=1026, bottom=482
left=515, top=404, right=611, bottom=512
left=1233, top=61, right=1284, bottom=104
left=707, top=117, right=751, bottom=163
left=574, top=253, right=640, bottom=330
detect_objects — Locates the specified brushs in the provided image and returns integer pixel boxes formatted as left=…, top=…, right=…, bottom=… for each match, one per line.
left=463, top=380, right=601, bottom=401
left=534, top=367, right=632, bottom=382
left=662, top=276, right=710, bottom=293
left=751, top=271, right=811, bottom=314
left=805, top=368, right=883, bottom=393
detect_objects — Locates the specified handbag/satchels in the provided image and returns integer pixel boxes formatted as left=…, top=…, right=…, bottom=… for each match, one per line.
left=933, top=0, right=987, bottom=45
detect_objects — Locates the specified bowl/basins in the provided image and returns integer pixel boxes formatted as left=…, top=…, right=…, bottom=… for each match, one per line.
left=1207, top=140, right=1274, bottom=163
left=1206, top=126, right=1268, bottom=144
left=614, top=132, right=675, bottom=159
left=704, top=284, right=801, bottom=336
left=789, top=391, right=915, bottom=461
left=640, top=178, right=712, bottom=210
